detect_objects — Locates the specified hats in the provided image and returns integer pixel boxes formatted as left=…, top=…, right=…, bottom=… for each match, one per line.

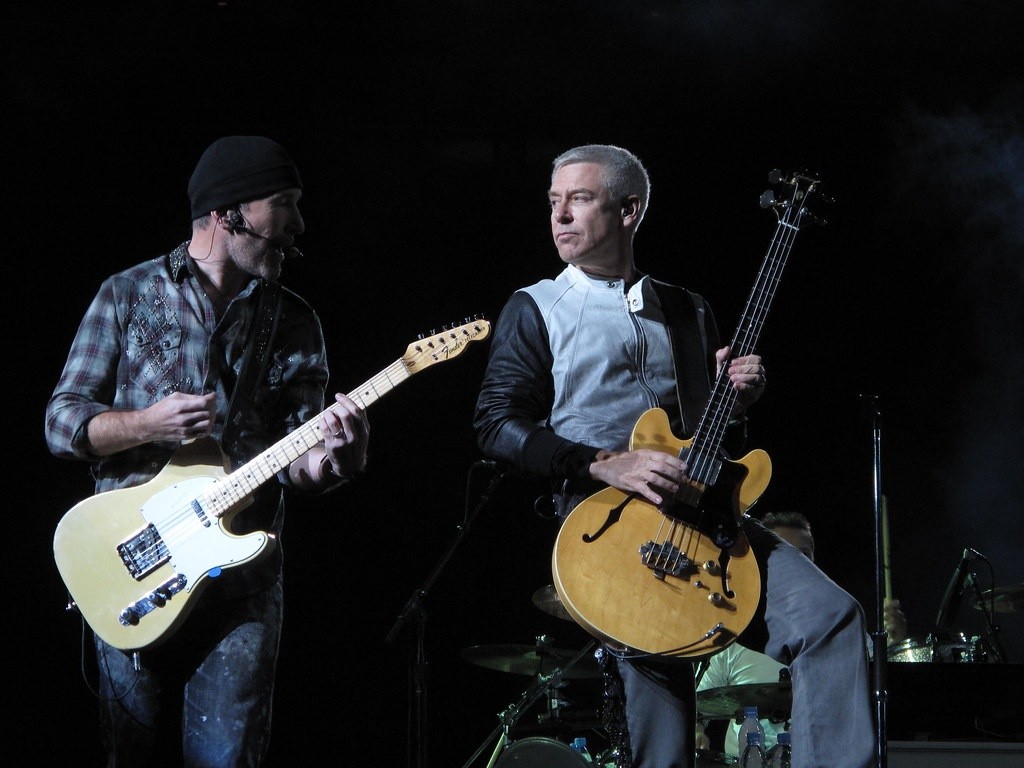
left=188, top=136, right=303, bottom=222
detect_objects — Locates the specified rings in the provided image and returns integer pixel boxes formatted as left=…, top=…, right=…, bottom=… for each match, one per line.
left=333, top=428, right=342, bottom=437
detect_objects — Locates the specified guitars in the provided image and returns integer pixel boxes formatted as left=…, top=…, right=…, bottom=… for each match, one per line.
left=53, top=313, right=494, bottom=658
left=549, top=165, right=839, bottom=664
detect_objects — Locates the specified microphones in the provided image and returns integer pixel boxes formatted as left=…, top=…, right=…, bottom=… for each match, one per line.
left=938, top=551, right=971, bottom=631
left=230, top=215, right=304, bottom=258
left=472, top=459, right=509, bottom=470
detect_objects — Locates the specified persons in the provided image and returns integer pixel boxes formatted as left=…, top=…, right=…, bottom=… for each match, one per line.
left=475, top=144, right=880, bottom=768
left=45, top=134, right=370, bottom=768
left=692, top=513, right=907, bottom=763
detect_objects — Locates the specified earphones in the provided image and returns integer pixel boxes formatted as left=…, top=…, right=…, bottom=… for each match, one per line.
left=623, top=205, right=634, bottom=216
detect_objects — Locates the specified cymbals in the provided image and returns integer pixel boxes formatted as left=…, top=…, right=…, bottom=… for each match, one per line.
left=463, top=643, right=599, bottom=681
left=697, top=682, right=792, bottom=719
